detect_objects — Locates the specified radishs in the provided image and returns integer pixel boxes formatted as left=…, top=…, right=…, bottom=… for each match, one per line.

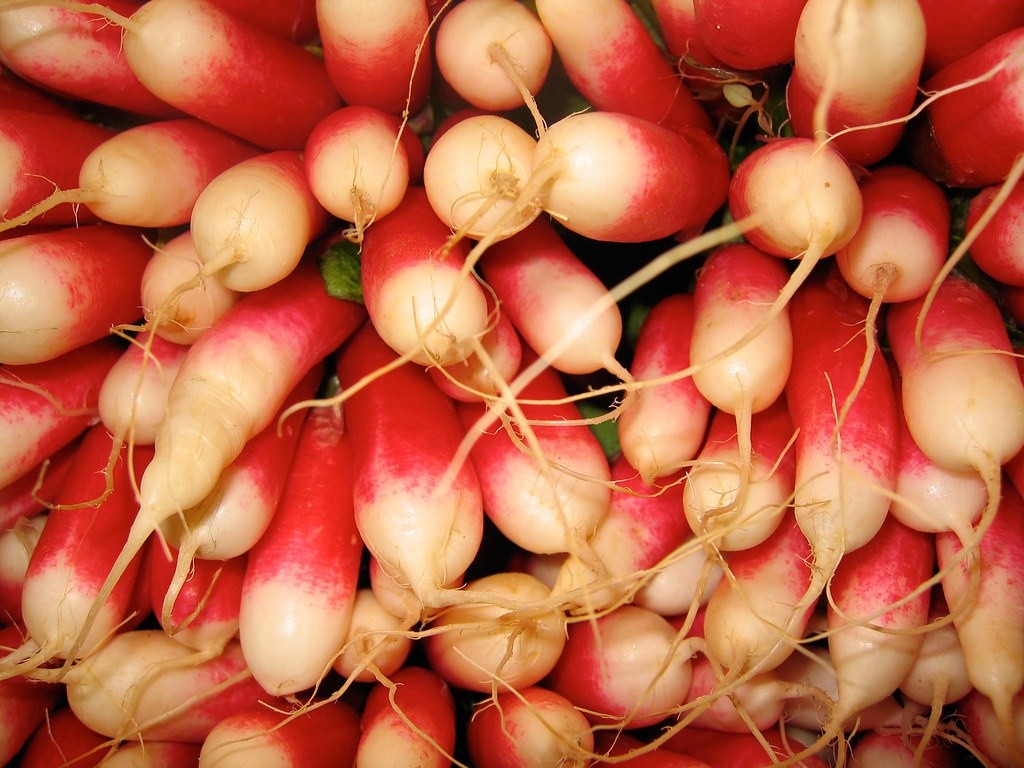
left=0, top=0, right=1023, bottom=768
